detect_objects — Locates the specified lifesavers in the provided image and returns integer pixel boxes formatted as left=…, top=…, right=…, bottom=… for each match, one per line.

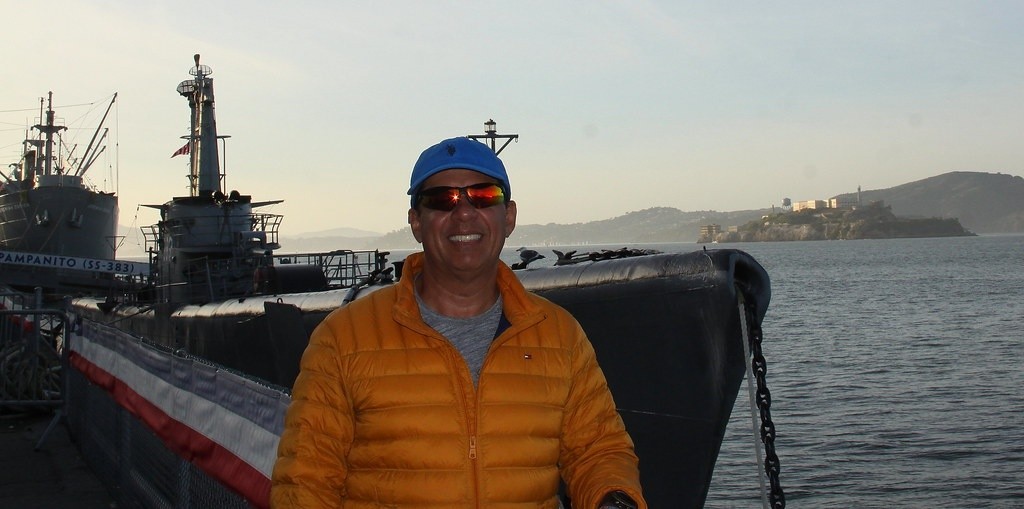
left=253, top=269, right=261, bottom=292
left=35, top=176, right=40, bottom=182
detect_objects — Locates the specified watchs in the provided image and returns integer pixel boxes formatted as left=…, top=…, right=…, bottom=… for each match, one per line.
left=604, top=491, right=638, bottom=509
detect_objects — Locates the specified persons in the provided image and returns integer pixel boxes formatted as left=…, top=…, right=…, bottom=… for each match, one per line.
left=270, top=135, right=649, bottom=509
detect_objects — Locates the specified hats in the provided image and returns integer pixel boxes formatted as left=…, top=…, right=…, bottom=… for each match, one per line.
left=406, top=136, right=511, bottom=208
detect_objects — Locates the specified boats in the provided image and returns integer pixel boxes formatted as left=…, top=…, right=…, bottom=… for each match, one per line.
left=65, top=51, right=774, bottom=509
left=0, top=91, right=119, bottom=291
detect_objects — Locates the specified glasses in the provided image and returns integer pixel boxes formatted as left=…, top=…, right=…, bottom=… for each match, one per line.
left=413, top=181, right=506, bottom=212
